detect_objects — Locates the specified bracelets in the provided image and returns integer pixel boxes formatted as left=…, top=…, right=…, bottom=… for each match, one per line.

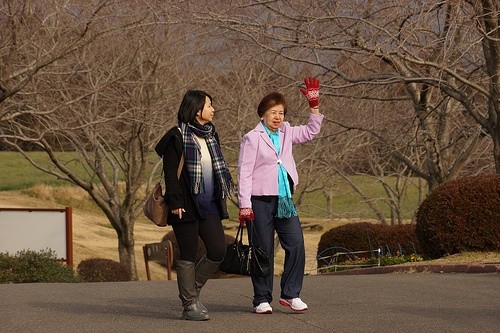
left=314, top=105, right=319, bottom=110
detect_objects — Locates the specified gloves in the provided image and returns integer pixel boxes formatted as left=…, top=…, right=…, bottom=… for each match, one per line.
left=238, top=208, right=255, bottom=227
left=300, top=77, right=320, bottom=108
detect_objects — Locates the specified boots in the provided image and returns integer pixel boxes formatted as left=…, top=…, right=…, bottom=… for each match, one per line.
left=175, top=259, right=210, bottom=321
left=195, top=252, right=226, bottom=314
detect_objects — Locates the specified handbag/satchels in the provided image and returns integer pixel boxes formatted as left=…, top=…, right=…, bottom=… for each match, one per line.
left=142, top=182, right=170, bottom=227
left=225, top=217, right=265, bottom=276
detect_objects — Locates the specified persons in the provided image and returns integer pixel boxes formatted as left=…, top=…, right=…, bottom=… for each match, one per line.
left=238, top=76, right=324, bottom=315
left=155, top=91, right=235, bottom=321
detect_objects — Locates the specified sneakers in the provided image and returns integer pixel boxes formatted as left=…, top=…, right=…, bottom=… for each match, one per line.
left=279, top=296, right=309, bottom=312
left=251, top=301, right=273, bottom=314
